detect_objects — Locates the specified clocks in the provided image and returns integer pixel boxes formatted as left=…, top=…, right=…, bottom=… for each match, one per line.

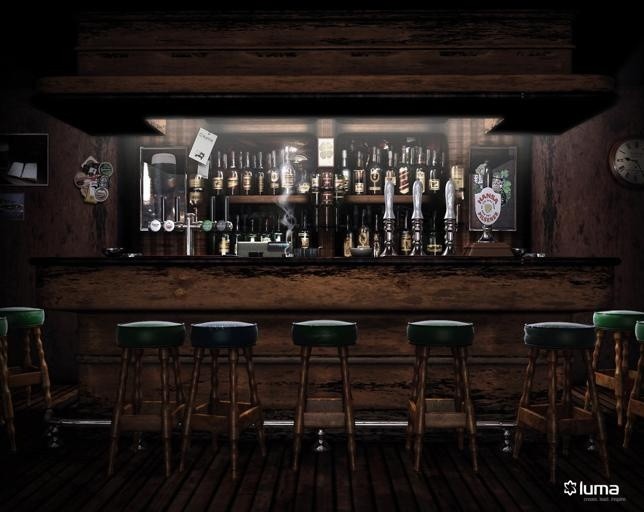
left=609, top=137, right=644, bottom=189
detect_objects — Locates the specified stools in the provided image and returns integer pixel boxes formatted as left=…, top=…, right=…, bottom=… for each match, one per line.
left=621, top=320, right=644, bottom=449
left=581, top=310, right=642, bottom=425
left=290, top=319, right=361, bottom=476
left=108, top=320, right=184, bottom=478
left=179, top=319, right=267, bottom=481
left=1, top=306, right=52, bottom=449
left=407, top=320, right=480, bottom=474
left=512, top=321, right=611, bottom=483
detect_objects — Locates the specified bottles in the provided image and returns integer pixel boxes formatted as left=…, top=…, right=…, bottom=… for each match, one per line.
left=416, top=147, right=427, bottom=195
left=339, top=149, right=352, bottom=194
left=410, top=148, right=416, bottom=183
left=231, top=214, right=243, bottom=254
left=236, top=151, right=243, bottom=170
left=366, top=147, right=383, bottom=195
left=352, top=205, right=359, bottom=232
left=260, top=218, right=272, bottom=243
left=426, top=150, right=442, bottom=195
left=217, top=196, right=232, bottom=255
left=383, top=150, right=399, bottom=184
left=189, top=173, right=204, bottom=220
left=343, top=214, right=355, bottom=257
left=274, top=214, right=284, bottom=242
left=439, top=151, right=448, bottom=184
left=353, top=151, right=367, bottom=196
left=242, top=152, right=254, bottom=195
left=281, top=145, right=296, bottom=194
left=254, top=152, right=267, bottom=196
left=211, top=150, right=225, bottom=195
left=393, top=152, right=399, bottom=169
left=406, top=146, right=410, bottom=166
left=267, top=150, right=282, bottom=195
left=372, top=213, right=382, bottom=256
left=226, top=150, right=240, bottom=195
left=208, top=196, right=219, bottom=254
left=454, top=204, right=463, bottom=254
left=397, top=144, right=411, bottom=195
left=400, top=209, right=413, bottom=256
left=358, top=208, right=371, bottom=247
left=297, top=209, right=312, bottom=249
left=251, top=154, right=258, bottom=176
left=245, top=218, right=257, bottom=241
left=265, top=153, right=271, bottom=170
left=426, top=148, right=431, bottom=167
left=223, top=153, right=228, bottom=169
left=367, top=206, right=372, bottom=227
left=427, top=209, right=443, bottom=256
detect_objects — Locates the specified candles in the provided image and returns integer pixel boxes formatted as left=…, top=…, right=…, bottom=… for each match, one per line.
left=301, top=238, right=309, bottom=247
